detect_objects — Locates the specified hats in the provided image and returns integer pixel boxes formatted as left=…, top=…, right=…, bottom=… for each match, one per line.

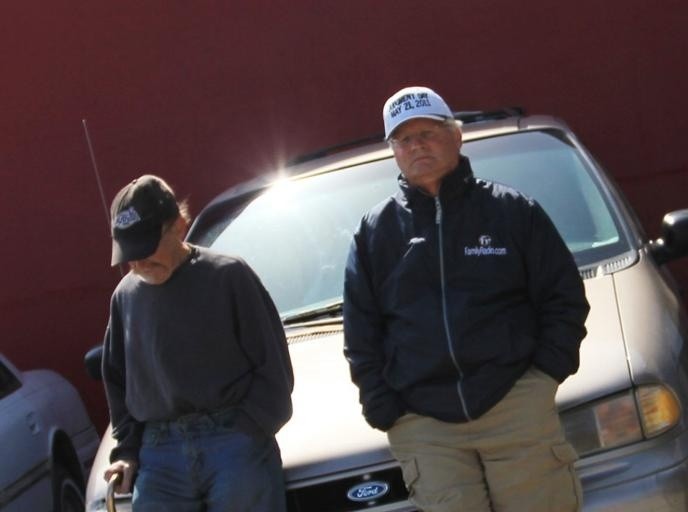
left=383, top=86, right=454, bottom=141
left=110, top=175, right=176, bottom=267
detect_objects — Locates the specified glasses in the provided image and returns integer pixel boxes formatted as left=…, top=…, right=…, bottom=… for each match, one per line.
left=393, top=129, right=449, bottom=143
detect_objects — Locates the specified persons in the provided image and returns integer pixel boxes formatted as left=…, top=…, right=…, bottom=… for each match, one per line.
left=101, top=174, right=294, bottom=512
left=343, top=85, right=590, bottom=512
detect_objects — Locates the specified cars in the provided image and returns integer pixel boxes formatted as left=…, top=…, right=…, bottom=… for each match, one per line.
left=81, top=108, right=688, bottom=512
left=0, top=353, right=102, bottom=512
